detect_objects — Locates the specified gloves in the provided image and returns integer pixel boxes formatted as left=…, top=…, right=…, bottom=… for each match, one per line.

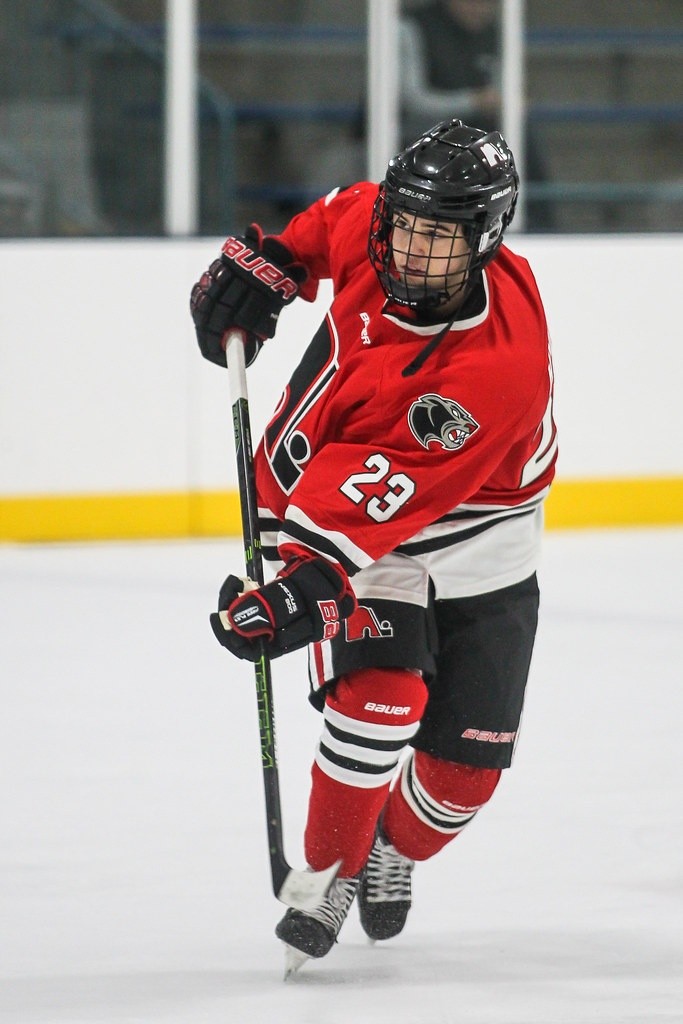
left=210, top=555, right=356, bottom=663
left=189, top=223, right=309, bottom=369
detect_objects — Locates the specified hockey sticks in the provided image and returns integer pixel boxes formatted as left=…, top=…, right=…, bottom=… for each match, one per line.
left=221, top=326, right=346, bottom=914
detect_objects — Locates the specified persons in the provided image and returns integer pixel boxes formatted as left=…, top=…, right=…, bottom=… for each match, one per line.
left=191, top=117, right=559, bottom=982
left=348, top=0, right=547, bottom=233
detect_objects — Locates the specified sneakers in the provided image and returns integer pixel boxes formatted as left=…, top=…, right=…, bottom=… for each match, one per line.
left=275, top=868, right=359, bottom=981
left=356, top=818, right=415, bottom=944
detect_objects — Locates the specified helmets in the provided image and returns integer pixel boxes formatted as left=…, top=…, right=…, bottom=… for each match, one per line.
left=367, top=118, right=520, bottom=310
